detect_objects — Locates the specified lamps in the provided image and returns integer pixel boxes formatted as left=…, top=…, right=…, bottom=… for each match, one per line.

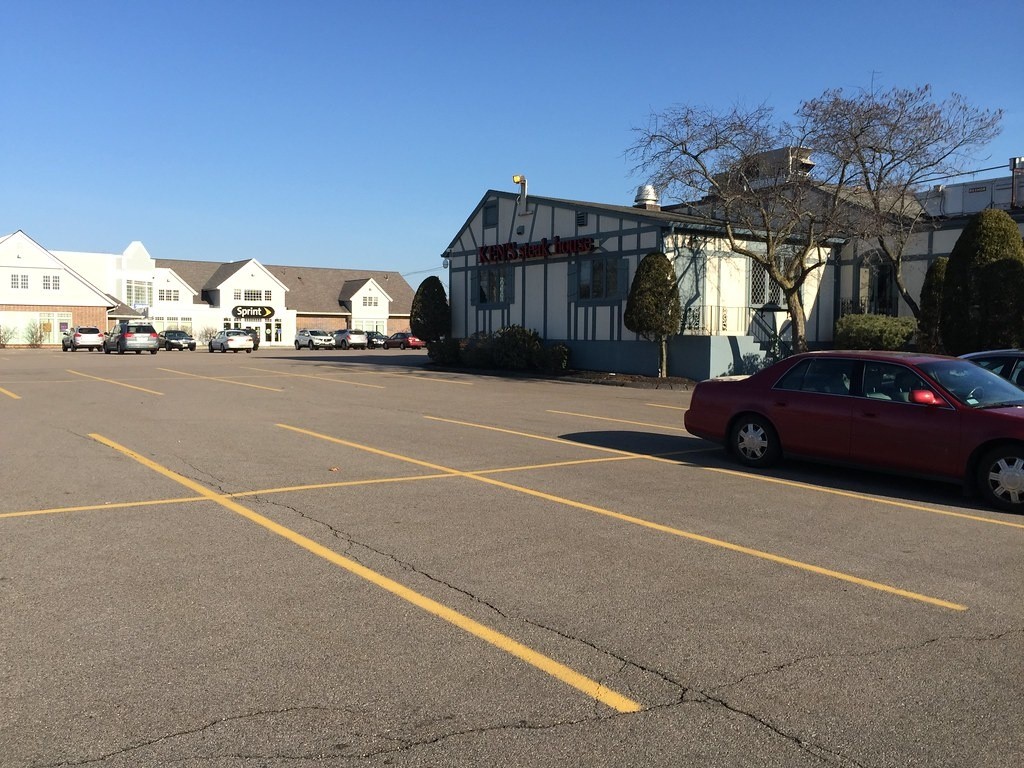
left=443, top=257, right=448, bottom=268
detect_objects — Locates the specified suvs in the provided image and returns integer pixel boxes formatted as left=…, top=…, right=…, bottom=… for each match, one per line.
left=103, top=322, right=159, bottom=355
left=62, top=326, right=104, bottom=352
left=295, top=329, right=367, bottom=351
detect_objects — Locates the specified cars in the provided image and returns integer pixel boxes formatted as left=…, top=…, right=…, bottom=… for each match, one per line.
left=685, top=348, right=1024, bottom=514
left=367, top=329, right=427, bottom=351
left=208, top=328, right=260, bottom=354
left=157, top=329, right=196, bottom=352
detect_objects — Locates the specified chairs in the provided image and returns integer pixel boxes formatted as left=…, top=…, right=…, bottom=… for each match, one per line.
left=894, top=373, right=913, bottom=402
left=865, top=371, right=891, bottom=400
